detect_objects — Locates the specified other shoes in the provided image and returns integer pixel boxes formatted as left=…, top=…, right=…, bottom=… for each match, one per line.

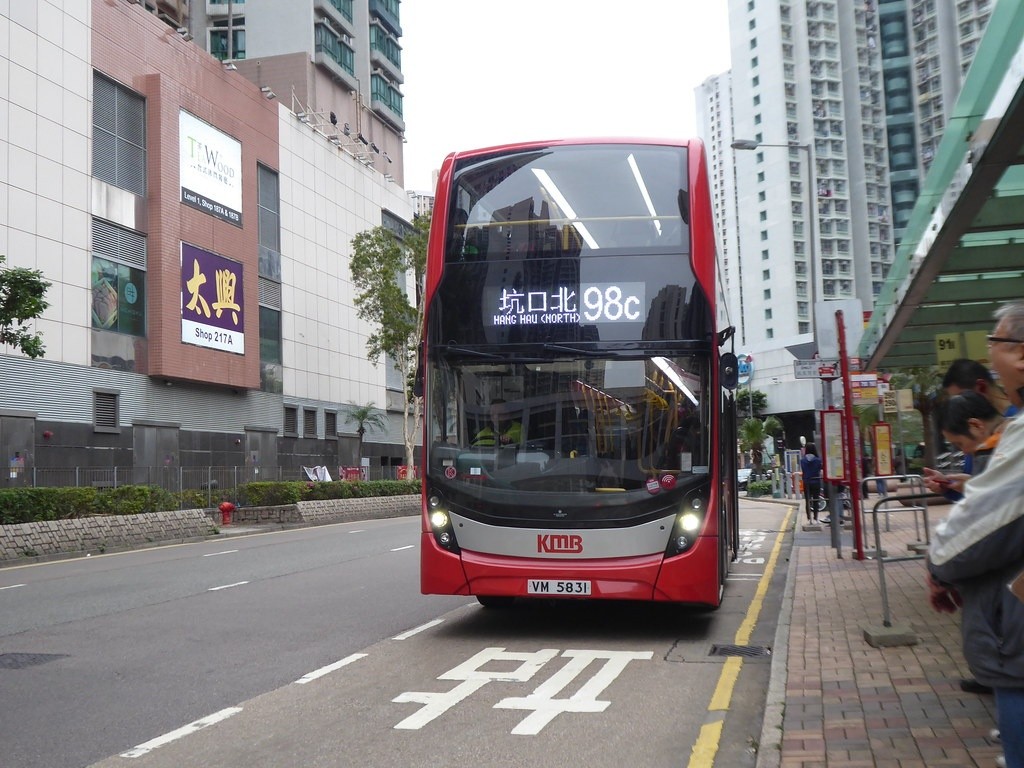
left=992, top=729, right=1002, bottom=743
left=995, top=755, right=1007, bottom=768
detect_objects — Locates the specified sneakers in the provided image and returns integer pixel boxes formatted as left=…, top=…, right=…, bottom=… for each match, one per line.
left=806, top=519, right=822, bottom=524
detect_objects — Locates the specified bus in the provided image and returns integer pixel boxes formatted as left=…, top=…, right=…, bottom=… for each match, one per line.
left=420, top=140, right=743, bottom=621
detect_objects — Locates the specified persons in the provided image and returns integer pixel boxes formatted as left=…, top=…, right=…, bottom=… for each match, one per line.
left=894, top=447, right=908, bottom=482
left=934, top=390, right=1016, bottom=766
left=472, top=399, right=526, bottom=450
left=820, top=478, right=846, bottom=525
left=862, top=453, right=873, bottom=498
left=925, top=303, right=1024, bottom=768
left=877, top=475, right=890, bottom=499
left=801, top=442, right=824, bottom=525
left=554, top=384, right=708, bottom=492
left=923, top=359, right=1021, bottom=694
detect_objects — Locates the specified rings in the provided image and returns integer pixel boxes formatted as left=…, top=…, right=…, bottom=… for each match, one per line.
left=505, top=439, right=508, bottom=440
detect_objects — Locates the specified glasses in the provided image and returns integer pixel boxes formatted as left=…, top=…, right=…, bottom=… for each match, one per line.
left=986, top=335, right=1024, bottom=346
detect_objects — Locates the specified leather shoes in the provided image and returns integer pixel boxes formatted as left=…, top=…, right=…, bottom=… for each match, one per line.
left=961, top=680, right=993, bottom=694
